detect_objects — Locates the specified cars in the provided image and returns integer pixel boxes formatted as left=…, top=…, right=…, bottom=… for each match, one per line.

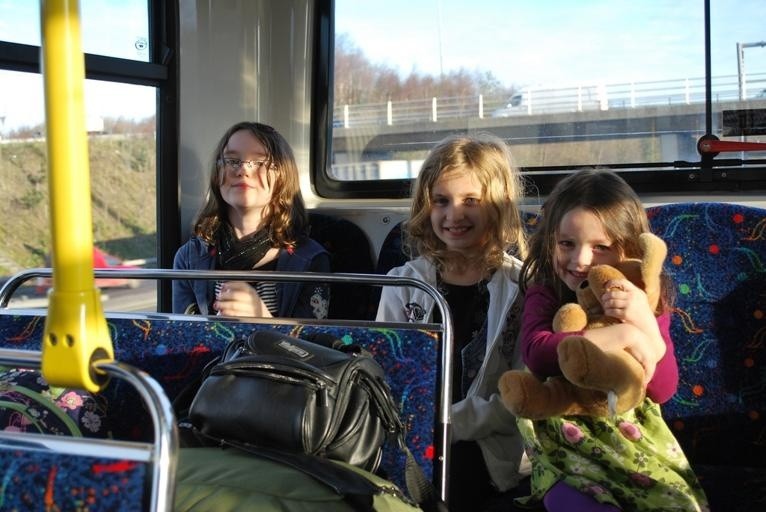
left=35, top=241, right=143, bottom=298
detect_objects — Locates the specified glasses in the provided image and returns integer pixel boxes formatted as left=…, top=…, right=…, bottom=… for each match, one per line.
left=218, top=157, right=276, bottom=171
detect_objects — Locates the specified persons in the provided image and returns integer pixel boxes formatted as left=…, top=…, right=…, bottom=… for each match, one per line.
left=514, top=165, right=715, bottom=512
left=170, top=119, right=335, bottom=320
left=370, top=130, right=538, bottom=511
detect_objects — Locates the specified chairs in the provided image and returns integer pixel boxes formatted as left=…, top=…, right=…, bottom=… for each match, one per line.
left=312, top=198, right=766, bottom=474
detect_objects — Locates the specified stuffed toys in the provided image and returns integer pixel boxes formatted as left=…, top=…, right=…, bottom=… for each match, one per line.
left=497, top=231, right=670, bottom=422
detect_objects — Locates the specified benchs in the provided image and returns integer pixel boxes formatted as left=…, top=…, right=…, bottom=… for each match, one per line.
left=1, top=265, right=451, bottom=512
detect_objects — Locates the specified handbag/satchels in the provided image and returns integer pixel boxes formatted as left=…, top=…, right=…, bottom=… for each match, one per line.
left=187, top=332, right=403, bottom=472
left=0, top=367, right=115, bottom=440
